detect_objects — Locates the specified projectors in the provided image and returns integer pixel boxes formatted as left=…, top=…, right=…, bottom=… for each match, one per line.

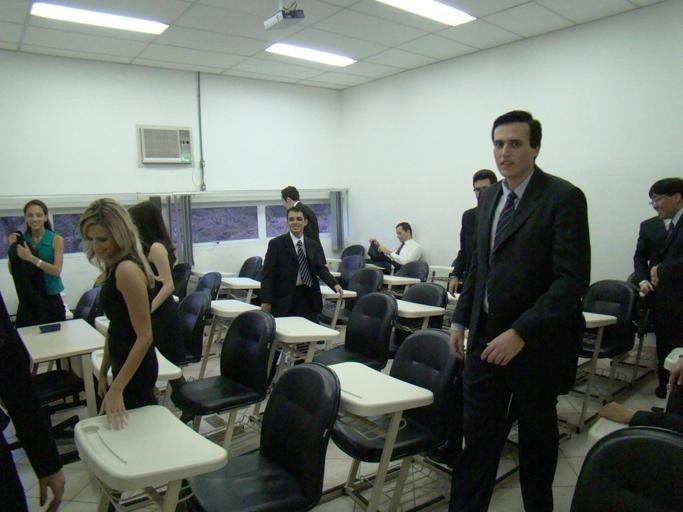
left=263, top=9, right=306, bottom=32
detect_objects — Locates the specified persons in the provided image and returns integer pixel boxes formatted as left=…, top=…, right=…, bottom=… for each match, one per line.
left=446, top=170, right=498, bottom=298
left=0, top=291, right=66, bottom=512
left=128, top=197, right=187, bottom=368
left=448, top=110, right=591, bottom=512
left=281, top=186, right=319, bottom=239
left=369, top=223, right=424, bottom=274
left=260, top=207, right=344, bottom=324
left=79, top=199, right=157, bottom=432
left=6, top=200, right=66, bottom=328
left=634, top=215, right=674, bottom=398
left=596, top=356, right=683, bottom=434
left=649, top=178, right=683, bottom=347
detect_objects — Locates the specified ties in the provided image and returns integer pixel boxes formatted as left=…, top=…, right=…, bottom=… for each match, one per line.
left=297, top=240, right=311, bottom=288
left=666, top=223, right=674, bottom=240
left=494, top=192, right=517, bottom=248
left=396, top=242, right=404, bottom=255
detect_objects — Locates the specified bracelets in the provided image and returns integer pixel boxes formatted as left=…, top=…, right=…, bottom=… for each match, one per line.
left=36, top=257, right=43, bottom=267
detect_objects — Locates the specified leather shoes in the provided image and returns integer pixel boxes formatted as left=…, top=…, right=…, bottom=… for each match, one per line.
left=656, top=386, right=667, bottom=398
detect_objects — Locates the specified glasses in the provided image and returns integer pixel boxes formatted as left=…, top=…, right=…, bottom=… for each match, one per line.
left=649, top=195, right=671, bottom=208
left=474, top=186, right=487, bottom=192
